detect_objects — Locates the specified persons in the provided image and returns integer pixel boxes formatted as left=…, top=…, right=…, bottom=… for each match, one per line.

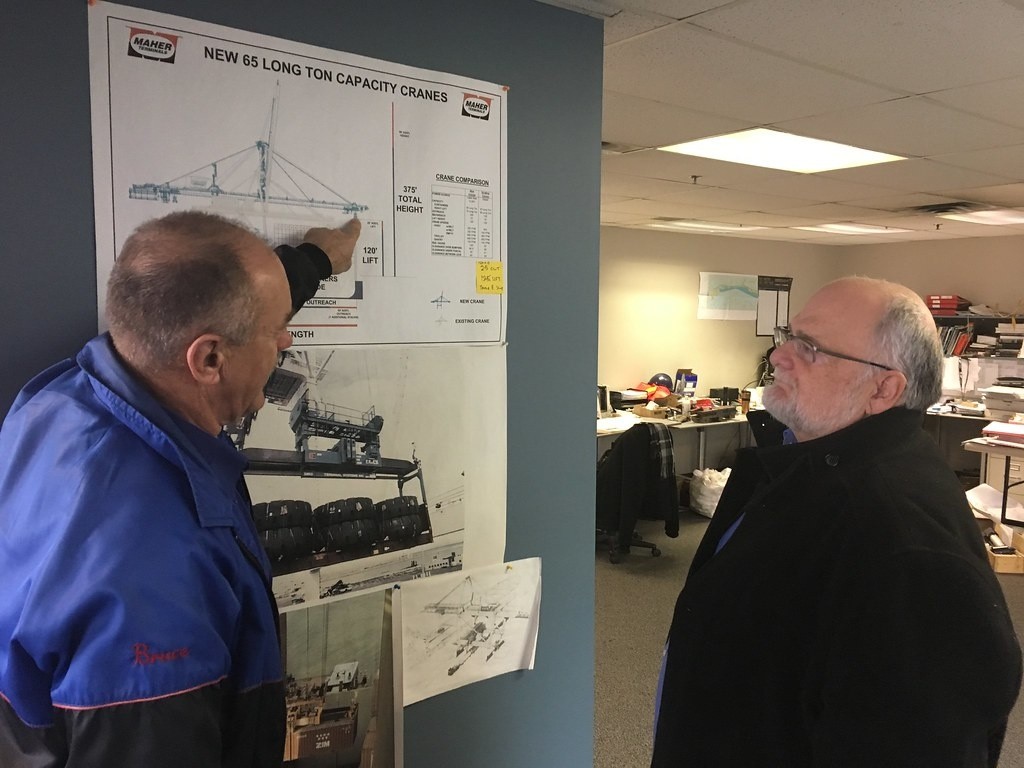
left=649, top=276, right=1024, bottom=768
left=0, top=210, right=361, bottom=768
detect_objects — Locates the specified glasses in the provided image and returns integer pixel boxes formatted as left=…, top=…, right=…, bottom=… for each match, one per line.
left=774, top=326, right=908, bottom=391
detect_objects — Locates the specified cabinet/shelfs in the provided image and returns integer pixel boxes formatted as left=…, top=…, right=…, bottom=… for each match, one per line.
left=937, top=412, right=1024, bottom=496
left=933, top=314, right=1024, bottom=360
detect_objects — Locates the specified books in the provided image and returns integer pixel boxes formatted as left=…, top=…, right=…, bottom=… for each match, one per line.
left=955, top=377, right=1024, bottom=449
left=938, top=323, right=1024, bottom=358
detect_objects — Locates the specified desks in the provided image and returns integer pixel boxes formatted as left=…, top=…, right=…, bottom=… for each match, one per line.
left=597, top=417, right=682, bottom=438
left=964, top=442, right=1024, bottom=528
left=668, top=414, right=749, bottom=470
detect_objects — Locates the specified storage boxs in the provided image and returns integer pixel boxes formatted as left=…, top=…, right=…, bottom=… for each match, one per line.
left=986, top=545, right=1024, bottom=574
left=674, top=368, right=697, bottom=397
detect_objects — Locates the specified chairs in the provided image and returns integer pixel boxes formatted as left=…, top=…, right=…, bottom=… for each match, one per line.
left=595, top=422, right=679, bottom=564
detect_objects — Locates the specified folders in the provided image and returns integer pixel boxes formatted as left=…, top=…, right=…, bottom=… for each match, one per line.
left=926, top=294, right=972, bottom=316
